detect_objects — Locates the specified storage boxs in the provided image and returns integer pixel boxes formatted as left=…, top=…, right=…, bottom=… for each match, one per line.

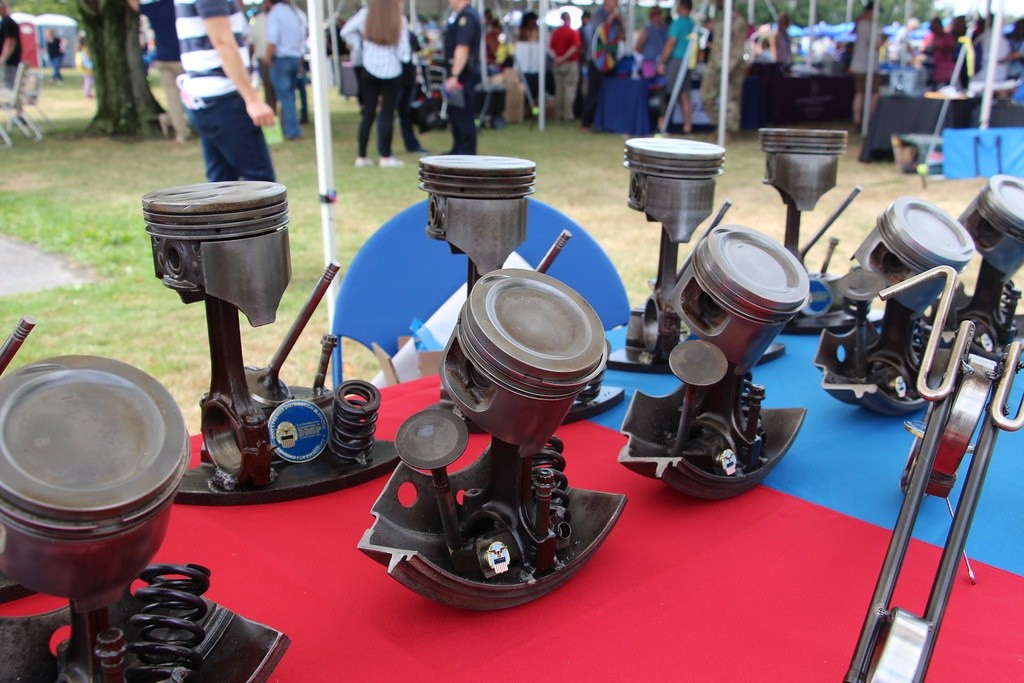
left=370, top=335, right=445, bottom=388
left=890, top=65, right=926, bottom=98
left=889, top=131, right=943, bottom=175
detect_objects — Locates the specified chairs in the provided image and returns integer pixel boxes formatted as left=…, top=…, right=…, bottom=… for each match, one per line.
left=332, top=196, right=630, bottom=392
left=423, top=64, right=451, bottom=124
left=0, top=60, right=59, bottom=144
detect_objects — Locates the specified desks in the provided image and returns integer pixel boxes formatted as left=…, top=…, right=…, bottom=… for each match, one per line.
left=0, top=320, right=1022, bottom=683
left=859, top=94, right=1024, bottom=161
left=667, top=73, right=858, bottom=131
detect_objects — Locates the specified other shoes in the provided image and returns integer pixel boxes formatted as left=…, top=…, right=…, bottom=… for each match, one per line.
left=355, top=158, right=373, bottom=166
left=379, top=157, right=403, bottom=167
left=13, top=118, right=26, bottom=126
left=409, top=149, right=429, bottom=155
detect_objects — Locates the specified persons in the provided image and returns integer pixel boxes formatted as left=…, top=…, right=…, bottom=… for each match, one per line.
left=0, top=0, right=1024, bottom=183
